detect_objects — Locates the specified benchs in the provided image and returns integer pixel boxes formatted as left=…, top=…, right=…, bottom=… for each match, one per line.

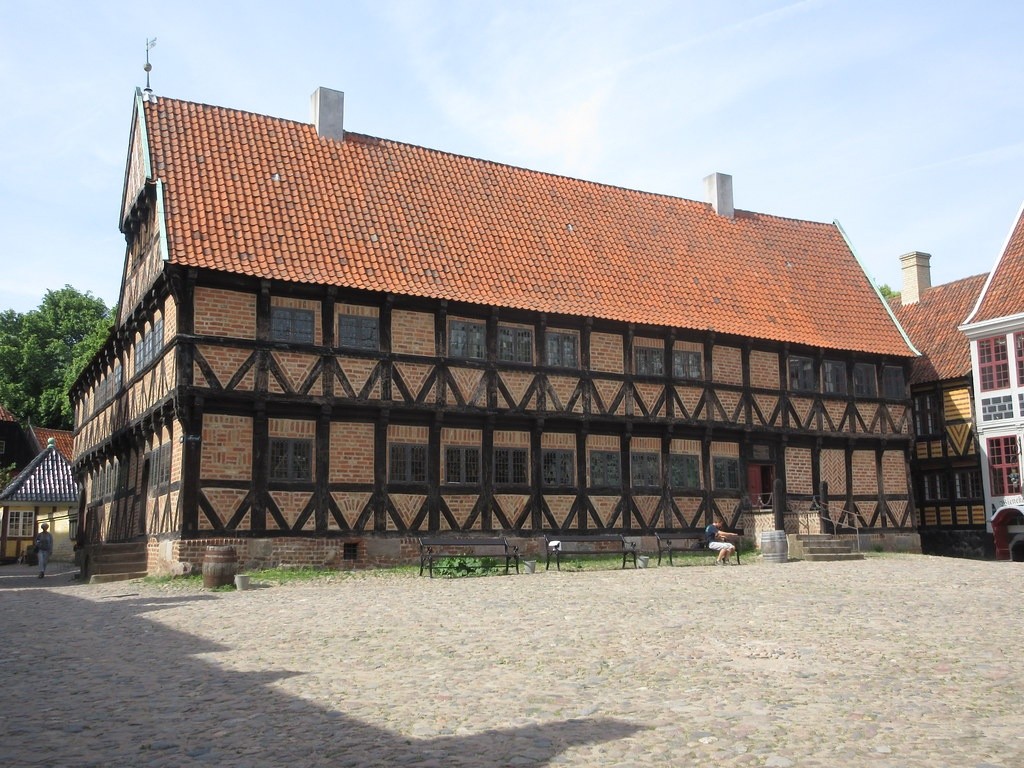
left=544, top=534, right=638, bottom=571
left=654, top=533, right=741, bottom=566
left=417, top=537, right=519, bottom=578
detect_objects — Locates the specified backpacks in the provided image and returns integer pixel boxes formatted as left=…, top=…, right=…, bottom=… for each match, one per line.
left=38, top=533, right=49, bottom=550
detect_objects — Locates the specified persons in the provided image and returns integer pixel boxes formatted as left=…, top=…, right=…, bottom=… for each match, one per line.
left=34, top=523, right=53, bottom=579
left=704, top=517, right=738, bottom=566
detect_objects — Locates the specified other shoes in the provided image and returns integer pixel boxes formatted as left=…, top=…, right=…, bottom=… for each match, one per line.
left=715, top=560, right=723, bottom=565
left=724, top=559, right=733, bottom=565
left=39, top=571, right=44, bottom=578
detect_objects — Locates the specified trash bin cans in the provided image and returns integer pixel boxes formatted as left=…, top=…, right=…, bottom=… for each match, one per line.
left=637, top=556, right=649, bottom=569
left=234, top=574, right=250, bottom=591
left=523, top=560, right=536, bottom=573
left=26, top=544, right=39, bottom=567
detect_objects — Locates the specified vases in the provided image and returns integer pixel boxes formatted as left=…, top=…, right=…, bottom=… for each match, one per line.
left=638, top=556, right=650, bottom=569
left=525, top=560, right=536, bottom=572
left=234, top=574, right=250, bottom=590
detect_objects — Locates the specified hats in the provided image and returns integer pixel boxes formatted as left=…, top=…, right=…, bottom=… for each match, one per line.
left=40, top=524, right=50, bottom=529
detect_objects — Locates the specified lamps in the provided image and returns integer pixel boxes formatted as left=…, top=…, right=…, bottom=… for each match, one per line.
left=1010, top=455, right=1019, bottom=484
